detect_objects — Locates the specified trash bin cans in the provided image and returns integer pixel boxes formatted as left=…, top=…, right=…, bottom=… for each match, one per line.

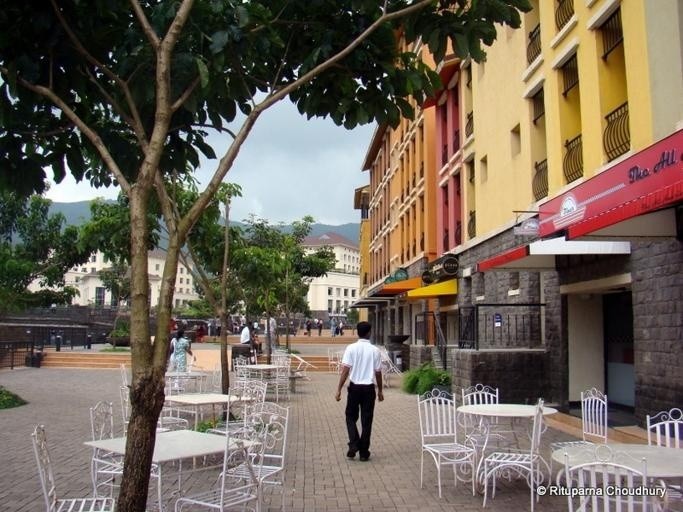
left=238, top=352, right=248, bottom=377
left=393, top=350, right=403, bottom=373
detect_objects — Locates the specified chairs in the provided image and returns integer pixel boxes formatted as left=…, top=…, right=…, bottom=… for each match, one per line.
left=417, top=383, right=683, bottom=512
left=30, top=345, right=404, bottom=512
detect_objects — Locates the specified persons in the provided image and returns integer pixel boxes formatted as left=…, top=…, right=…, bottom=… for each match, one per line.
left=334, top=320, right=384, bottom=462
left=169, top=329, right=196, bottom=372
left=0, top=300, right=343, bottom=349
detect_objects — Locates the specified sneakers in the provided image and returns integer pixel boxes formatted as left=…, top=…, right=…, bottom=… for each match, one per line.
left=348, top=443, right=359, bottom=457
left=360, top=451, right=370, bottom=461
